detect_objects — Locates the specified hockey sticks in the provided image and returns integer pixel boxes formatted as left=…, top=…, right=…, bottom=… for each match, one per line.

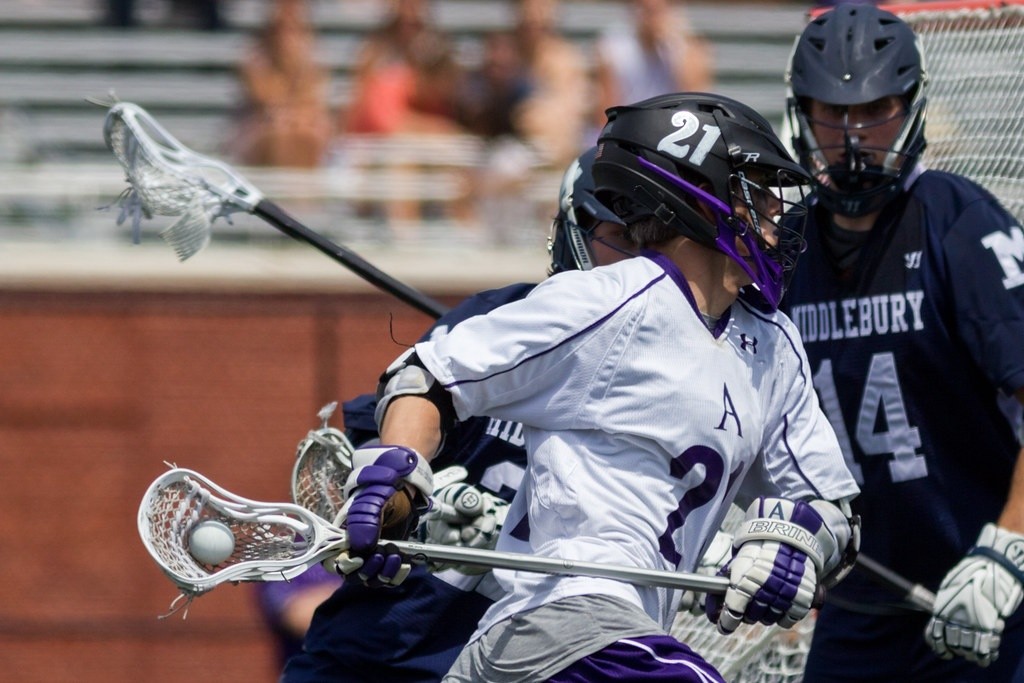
left=98, top=100, right=936, bottom=612
left=285, top=422, right=473, bottom=530
left=136, top=456, right=731, bottom=624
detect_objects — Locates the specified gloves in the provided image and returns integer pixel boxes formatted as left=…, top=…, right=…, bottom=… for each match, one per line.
left=426, top=482, right=510, bottom=577
left=700, top=498, right=852, bottom=637
left=334, top=445, right=433, bottom=591
left=922, top=522, right=1024, bottom=667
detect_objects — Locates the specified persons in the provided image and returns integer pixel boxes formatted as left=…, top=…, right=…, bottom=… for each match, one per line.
left=233, top=0, right=713, bottom=167
left=257, top=93, right=861, bottom=683
left=777, top=4, right=1024, bottom=683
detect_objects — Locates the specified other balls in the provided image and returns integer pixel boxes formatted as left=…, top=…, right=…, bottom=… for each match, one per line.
left=185, top=518, right=235, bottom=566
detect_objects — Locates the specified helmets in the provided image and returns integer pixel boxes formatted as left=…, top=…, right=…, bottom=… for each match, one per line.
left=593, top=90, right=818, bottom=320
left=780, top=3, right=929, bottom=219
left=544, top=145, right=649, bottom=280
